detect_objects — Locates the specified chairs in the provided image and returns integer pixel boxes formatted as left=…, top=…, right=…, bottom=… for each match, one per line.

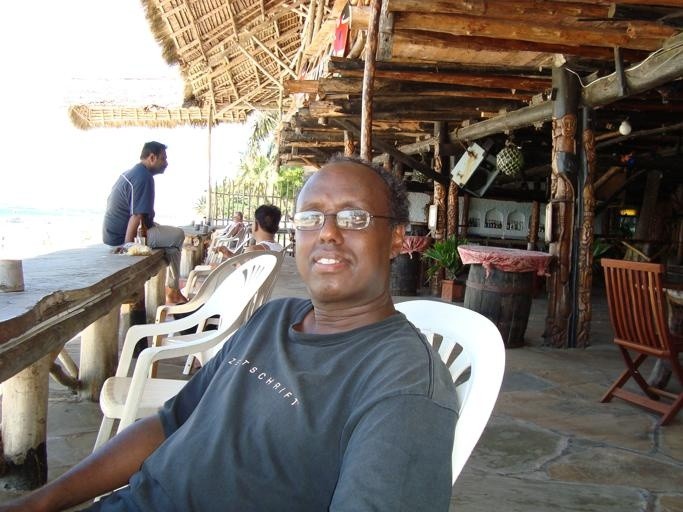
left=394, top=300, right=506, bottom=487
left=599, top=258, right=682, bottom=426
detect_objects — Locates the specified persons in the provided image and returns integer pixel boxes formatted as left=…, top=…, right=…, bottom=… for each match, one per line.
left=172, top=202, right=282, bottom=369
left=101, top=141, right=188, bottom=305
left=0, top=154, right=460, bottom=512
left=224, top=211, right=244, bottom=248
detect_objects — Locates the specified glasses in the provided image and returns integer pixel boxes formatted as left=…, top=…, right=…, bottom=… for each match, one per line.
left=290, top=206, right=408, bottom=233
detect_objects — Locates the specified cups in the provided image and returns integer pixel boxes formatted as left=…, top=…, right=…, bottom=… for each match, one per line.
left=133, top=237, right=145, bottom=246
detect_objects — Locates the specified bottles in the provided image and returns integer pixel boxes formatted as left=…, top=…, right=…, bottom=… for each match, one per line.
left=466, top=216, right=545, bottom=233
left=136, top=219, right=147, bottom=246
left=191, top=216, right=211, bottom=235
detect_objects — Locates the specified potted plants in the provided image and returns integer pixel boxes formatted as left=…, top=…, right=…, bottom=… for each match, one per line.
left=422, top=233, right=474, bottom=302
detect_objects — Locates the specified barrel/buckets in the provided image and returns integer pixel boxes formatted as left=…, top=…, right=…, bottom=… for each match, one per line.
left=389, top=251, right=421, bottom=295
left=463, top=263, right=532, bottom=349
